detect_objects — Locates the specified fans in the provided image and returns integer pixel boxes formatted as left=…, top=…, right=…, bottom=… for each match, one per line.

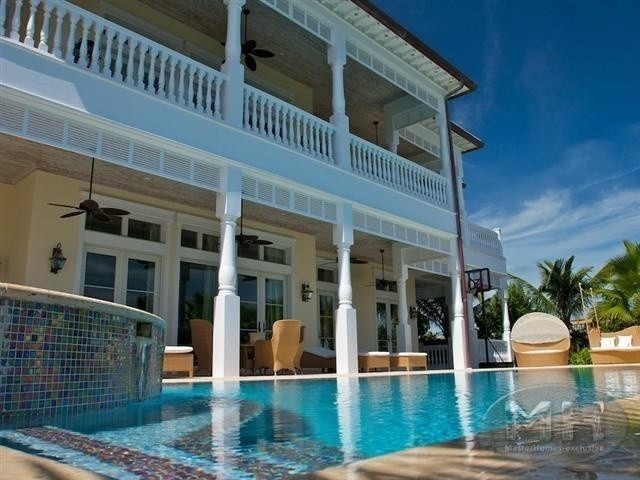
left=220, top=8, right=275, bottom=72
left=235, top=198, right=273, bottom=249
left=47, top=157, right=131, bottom=223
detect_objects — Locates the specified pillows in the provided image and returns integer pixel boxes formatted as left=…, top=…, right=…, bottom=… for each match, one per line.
left=600, top=336, right=633, bottom=350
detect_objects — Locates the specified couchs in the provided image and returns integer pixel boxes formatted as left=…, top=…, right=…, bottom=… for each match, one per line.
left=588, top=326, right=640, bottom=365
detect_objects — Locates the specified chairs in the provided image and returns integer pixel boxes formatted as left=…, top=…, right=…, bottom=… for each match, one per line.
left=164, top=318, right=307, bottom=378
left=301, top=347, right=429, bottom=373
left=510, top=312, right=571, bottom=367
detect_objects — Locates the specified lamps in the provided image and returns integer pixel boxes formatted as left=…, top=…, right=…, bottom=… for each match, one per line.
left=410, top=305, right=417, bottom=319
left=49, top=241, right=67, bottom=275
left=302, top=280, right=314, bottom=304
